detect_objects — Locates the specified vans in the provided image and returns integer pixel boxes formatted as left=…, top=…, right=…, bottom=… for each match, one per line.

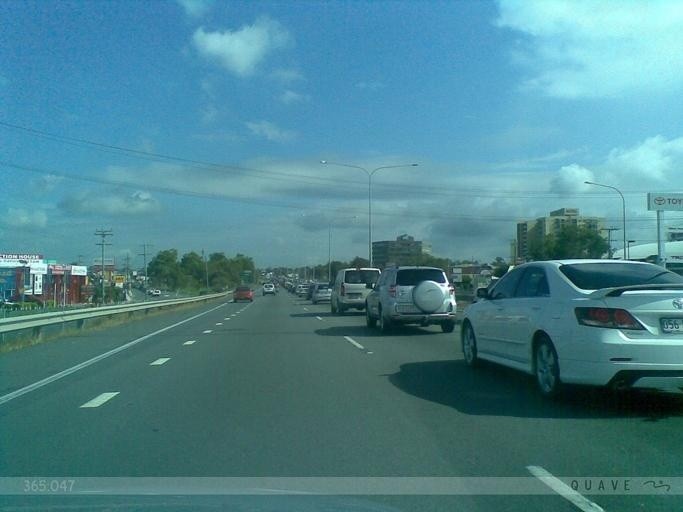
left=331, top=265, right=385, bottom=316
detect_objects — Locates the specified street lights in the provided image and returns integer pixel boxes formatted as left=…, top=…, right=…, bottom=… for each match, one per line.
left=319, top=160, right=420, bottom=267
left=299, top=213, right=361, bottom=282
left=582, top=181, right=627, bottom=259
left=627, top=240, right=635, bottom=260
left=18, top=258, right=28, bottom=308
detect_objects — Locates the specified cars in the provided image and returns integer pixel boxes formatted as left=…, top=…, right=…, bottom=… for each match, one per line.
left=457, top=258, right=682, bottom=403
left=128, top=279, right=162, bottom=298
left=231, top=271, right=332, bottom=305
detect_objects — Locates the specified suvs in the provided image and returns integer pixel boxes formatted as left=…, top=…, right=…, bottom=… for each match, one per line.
left=362, top=263, right=458, bottom=335
